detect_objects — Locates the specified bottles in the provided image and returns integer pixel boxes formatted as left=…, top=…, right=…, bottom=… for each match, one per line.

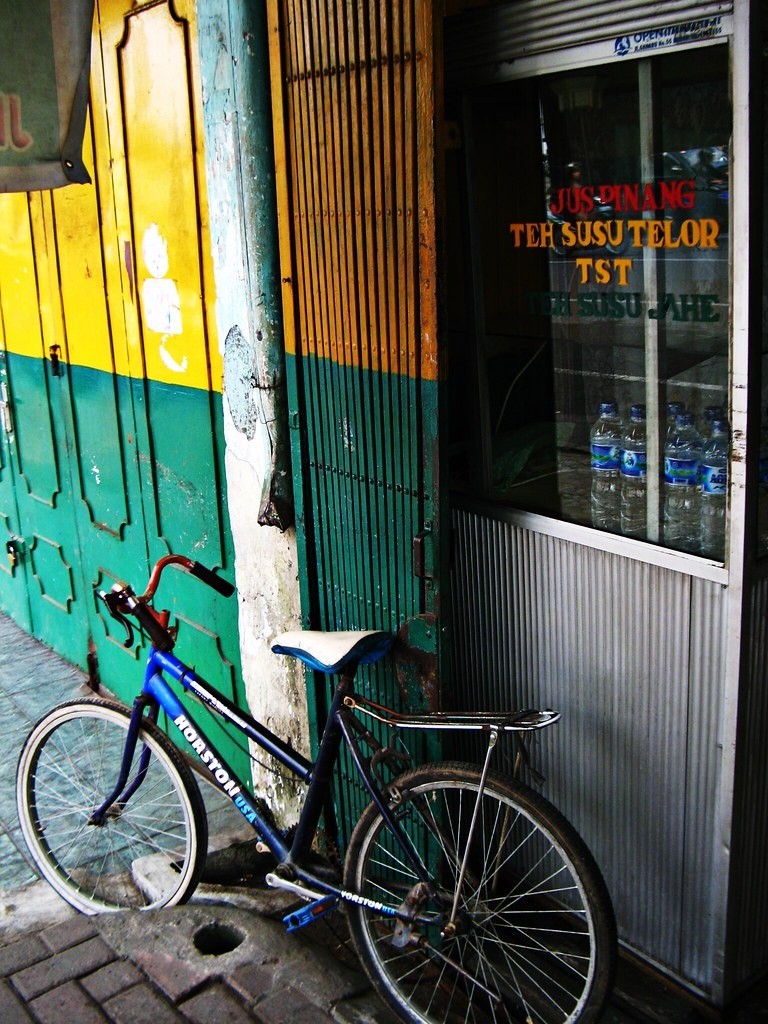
left=620, top=401, right=686, bottom=536
left=663, top=406, right=731, bottom=561
left=588, top=400, right=626, bottom=532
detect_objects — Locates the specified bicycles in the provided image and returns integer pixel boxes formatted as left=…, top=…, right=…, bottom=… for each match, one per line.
left=11, top=553, right=620, bottom=1024
